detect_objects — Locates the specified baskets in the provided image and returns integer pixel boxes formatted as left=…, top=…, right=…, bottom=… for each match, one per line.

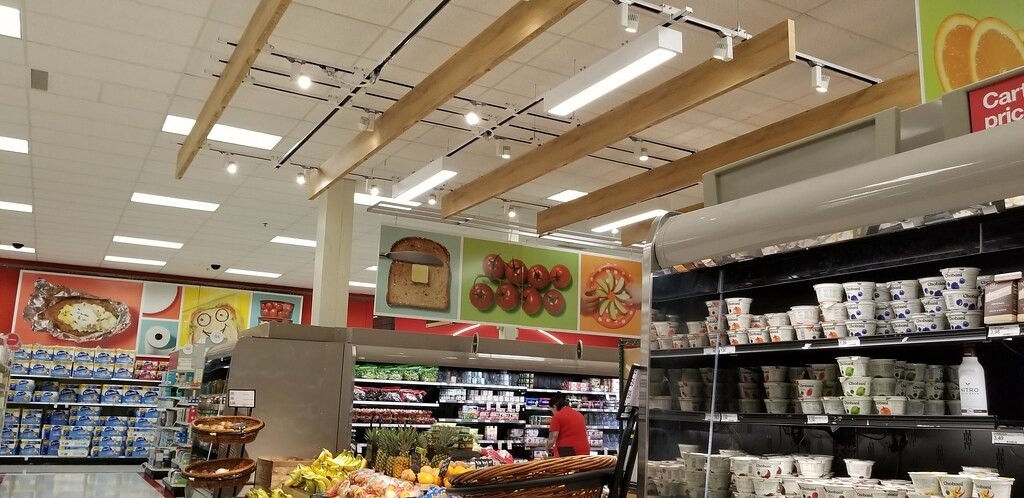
left=447, top=455, right=617, bottom=498
left=191, top=415, right=265, bottom=444
left=181, top=458, right=256, bottom=488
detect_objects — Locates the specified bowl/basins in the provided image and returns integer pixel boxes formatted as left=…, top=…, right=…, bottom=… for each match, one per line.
left=649, top=356, right=962, bottom=415
left=649, top=267, right=984, bottom=349
left=260, top=300, right=295, bottom=319
left=647, top=443, right=1016, bottom=498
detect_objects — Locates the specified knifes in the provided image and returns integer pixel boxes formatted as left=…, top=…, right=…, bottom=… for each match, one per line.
left=379, top=251, right=444, bottom=266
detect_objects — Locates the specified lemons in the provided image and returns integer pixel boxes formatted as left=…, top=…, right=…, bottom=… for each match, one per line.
left=398, top=460, right=473, bottom=487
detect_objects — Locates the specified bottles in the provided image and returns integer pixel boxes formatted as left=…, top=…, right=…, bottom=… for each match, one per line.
left=189, top=405, right=196, bottom=423
left=958, top=350, right=989, bottom=417
left=206, top=379, right=225, bottom=394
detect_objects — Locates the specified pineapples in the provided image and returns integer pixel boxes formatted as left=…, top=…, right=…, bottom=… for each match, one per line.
left=364, top=425, right=455, bottom=477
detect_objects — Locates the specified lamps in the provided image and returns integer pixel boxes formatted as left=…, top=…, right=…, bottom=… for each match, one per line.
left=805, top=60, right=831, bottom=93
left=633, top=140, right=648, bottom=162
left=496, top=139, right=510, bottom=159
left=288, top=60, right=310, bottom=89
left=391, top=141, right=458, bottom=204
left=713, top=29, right=734, bottom=62
left=360, top=110, right=375, bottom=131
left=504, top=201, right=516, bottom=218
left=616, top=1, right=639, bottom=34
left=544, top=25, right=683, bottom=116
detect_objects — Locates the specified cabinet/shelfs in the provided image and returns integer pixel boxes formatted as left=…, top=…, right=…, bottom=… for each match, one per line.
left=141, top=339, right=341, bottom=498
left=348, top=378, right=620, bottom=462
left=640, top=202, right=1024, bottom=498
left=0, top=344, right=168, bottom=464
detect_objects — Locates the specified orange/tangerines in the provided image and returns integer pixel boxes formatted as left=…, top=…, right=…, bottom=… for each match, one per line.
left=934, top=12, right=1024, bottom=94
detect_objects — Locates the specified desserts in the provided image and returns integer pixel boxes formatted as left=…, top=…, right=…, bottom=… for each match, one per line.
left=45, top=297, right=119, bottom=338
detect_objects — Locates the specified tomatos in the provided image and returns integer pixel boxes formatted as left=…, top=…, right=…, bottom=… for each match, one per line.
left=495, top=283, right=518, bottom=311
left=519, top=286, right=542, bottom=314
left=504, top=258, right=528, bottom=285
left=469, top=283, right=494, bottom=310
left=550, top=265, right=572, bottom=289
left=527, top=264, right=549, bottom=290
left=544, top=290, right=563, bottom=314
left=482, top=253, right=504, bottom=281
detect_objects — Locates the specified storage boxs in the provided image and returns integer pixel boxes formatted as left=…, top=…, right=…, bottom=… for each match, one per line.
left=3, top=344, right=164, bottom=460
left=484, top=426, right=497, bottom=441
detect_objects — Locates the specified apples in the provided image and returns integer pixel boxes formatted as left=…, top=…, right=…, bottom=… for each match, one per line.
left=594, top=272, right=631, bottom=320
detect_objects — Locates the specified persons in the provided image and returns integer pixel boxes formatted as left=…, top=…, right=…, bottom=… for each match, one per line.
left=546, top=392, right=591, bottom=458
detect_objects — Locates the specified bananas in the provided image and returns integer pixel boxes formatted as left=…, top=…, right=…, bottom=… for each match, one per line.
left=244, top=448, right=368, bottom=498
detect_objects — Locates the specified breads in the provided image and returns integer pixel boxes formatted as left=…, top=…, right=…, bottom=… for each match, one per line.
left=189, top=305, right=238, bottom=347
left=386, top=237, right=450, bottom=311
